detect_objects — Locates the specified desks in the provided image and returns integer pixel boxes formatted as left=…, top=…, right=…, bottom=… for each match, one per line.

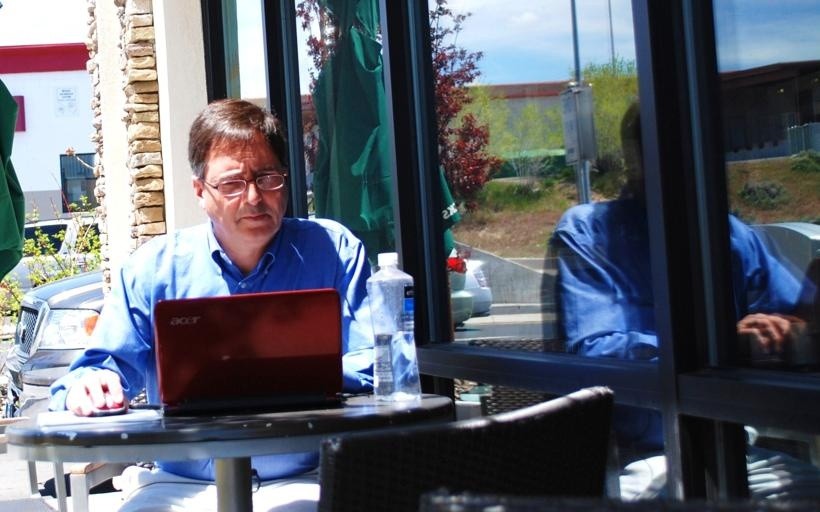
left=6, top=391, right=458, bottom=512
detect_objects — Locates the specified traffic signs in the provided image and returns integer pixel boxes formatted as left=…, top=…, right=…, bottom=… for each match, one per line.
left=559, top=87, right=583, bottom=166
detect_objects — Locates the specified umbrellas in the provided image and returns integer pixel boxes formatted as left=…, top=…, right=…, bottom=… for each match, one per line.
left=0, top=77, right=26, bottom=287
left=312, top=2, right=462, bottom=274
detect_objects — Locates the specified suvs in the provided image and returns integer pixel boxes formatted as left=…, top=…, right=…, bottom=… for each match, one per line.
left=7, top=266, right=109, bottom=409
left=12, top=216, right=101, bottom=290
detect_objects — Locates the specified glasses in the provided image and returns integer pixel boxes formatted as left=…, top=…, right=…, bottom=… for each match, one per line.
left=203, top=171, right=288, bottom=198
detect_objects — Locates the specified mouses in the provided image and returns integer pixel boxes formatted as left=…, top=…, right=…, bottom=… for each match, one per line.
left=91, top=392, right=129, bottom=417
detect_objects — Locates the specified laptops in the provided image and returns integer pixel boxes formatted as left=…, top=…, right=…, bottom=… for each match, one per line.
left=153, top=287, right=345, bottom=417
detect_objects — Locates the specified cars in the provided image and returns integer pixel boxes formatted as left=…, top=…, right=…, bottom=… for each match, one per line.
left=462, top=258, right=492, bottom=318
left=443, top=247, right=473, bottom=331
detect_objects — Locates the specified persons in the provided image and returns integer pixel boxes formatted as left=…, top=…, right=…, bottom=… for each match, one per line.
left=539, top=100, right=819, bottom=505
left=46, top=98, right=420, bottom=512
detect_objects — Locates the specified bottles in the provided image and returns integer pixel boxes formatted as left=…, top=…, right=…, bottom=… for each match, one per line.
left=368, top=253, right=425, bottom=401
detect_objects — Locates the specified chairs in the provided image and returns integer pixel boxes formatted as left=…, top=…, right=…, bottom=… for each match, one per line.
left=315, top=385, right=613, bottom=512
